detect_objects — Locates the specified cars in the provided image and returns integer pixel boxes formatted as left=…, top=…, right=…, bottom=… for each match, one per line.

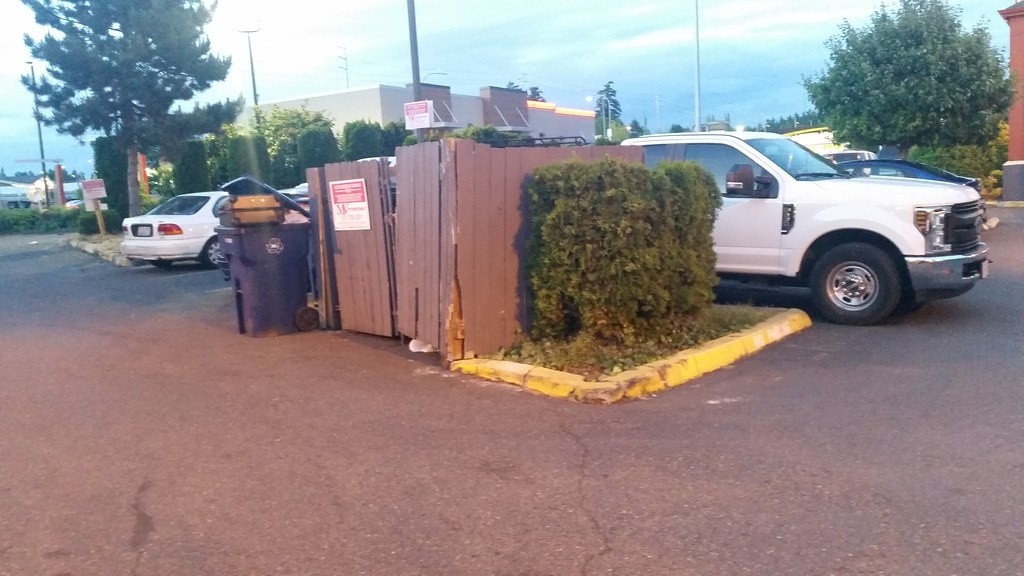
left=120, top=191, right=230, bottom=267
left=820, top=151, right=877, bottom=176
left=621, top=130, right=993, bottom=326
left=278, top=177, right=396, bottom=222
left=357, top=156, right=397, bottom=168
left=66, top=200, right=84, bottom=208
left=837, top=159, right=982, bottom=199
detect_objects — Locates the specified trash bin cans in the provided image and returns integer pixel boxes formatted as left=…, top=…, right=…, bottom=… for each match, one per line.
left=1002, top=159, right=1024, bottom=201
left=8, top=201, right=19, bottom=209
left=20, top=201, right=31, bottom=209
left=213, top=176, right=320, bottom=340
